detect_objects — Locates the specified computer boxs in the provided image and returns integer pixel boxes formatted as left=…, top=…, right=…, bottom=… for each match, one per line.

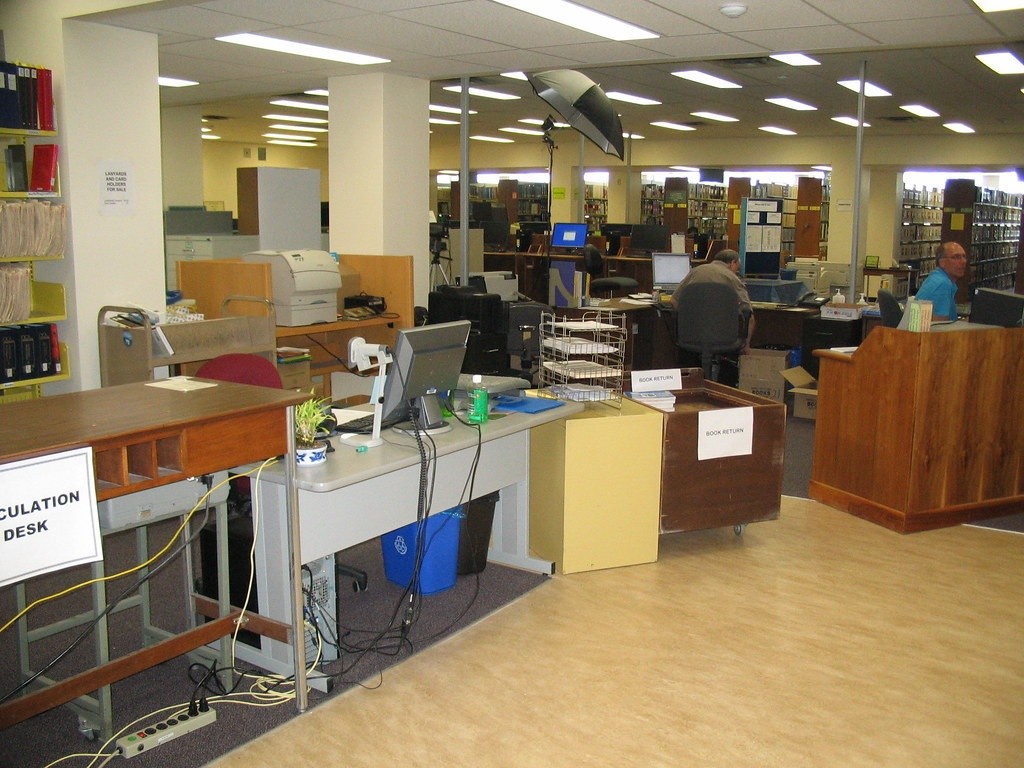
left=200, top=518, right=339, bottom=669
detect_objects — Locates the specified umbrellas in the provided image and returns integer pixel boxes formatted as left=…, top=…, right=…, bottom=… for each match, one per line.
left=525, top=69, right=624, bottom=163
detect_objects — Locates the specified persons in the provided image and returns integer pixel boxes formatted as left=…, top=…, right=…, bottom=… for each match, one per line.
left=671, top=248, right=756, bottom=385
left=914, top=242, right=972, bottom=324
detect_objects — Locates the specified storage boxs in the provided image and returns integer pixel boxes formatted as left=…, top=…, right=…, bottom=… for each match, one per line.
left=779, top=366, right=819, bottom=419
left=862, top=306, right=880, bottom=316
left=277, top=361, right=311, bottom=389
left=737, top=348, right=790, bottom=403
left=819, top=303, right=863, bottom=319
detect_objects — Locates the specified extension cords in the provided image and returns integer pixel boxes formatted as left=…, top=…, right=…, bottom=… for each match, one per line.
left=114, top=705, right=217, bottom=758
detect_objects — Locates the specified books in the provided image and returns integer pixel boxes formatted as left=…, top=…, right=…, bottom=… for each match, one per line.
left=1, top=59, right=65, bottom=384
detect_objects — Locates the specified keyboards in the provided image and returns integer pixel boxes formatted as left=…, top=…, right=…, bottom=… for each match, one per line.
left=335, top=413, right=419, bottom=434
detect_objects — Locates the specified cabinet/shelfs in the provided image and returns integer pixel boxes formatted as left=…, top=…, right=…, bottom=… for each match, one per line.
left=802, top=313, right=863, bottom=381
left=97, top=293, right=278, bottom=388
left=236, top=167, right=321, bottom=250
left=525, top=388, right=663, bottom=577
left=0, top=59, right=69, bottom=404
left=436, top=175, right=1024, bottom=306
left=537, top=309, right=627, bottom=411
left=165, top=235, right=261, bottom=291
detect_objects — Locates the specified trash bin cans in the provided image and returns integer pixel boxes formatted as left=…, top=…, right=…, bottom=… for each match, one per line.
left=765, top=343, right=802, bottom=398
left=457, top=489, right=501, bottom=575
left=382, top=506, right=462, bottom=595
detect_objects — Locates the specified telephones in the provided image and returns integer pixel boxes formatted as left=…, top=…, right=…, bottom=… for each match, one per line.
left=798, top=292, right=830, bottom=308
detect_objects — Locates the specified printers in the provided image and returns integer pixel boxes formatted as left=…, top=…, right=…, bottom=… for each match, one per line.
left=241, top=249, right=342, bottom=327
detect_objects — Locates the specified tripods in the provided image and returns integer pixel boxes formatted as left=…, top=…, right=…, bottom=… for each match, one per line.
left=429, top=238, right=452, bottom=293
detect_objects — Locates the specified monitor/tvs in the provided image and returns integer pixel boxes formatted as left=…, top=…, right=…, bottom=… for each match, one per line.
left=628, top=224, right=671, bottom=255
left=968, top=287, right=1024, bottom=328
left=551, top=223, right=589, bottom=254
left=600, top=223, right=632, bottom=255
left=652, top=253, right=692, bottom=295
left=518, top=221, right=550, bottom=248
left=380, top=320, right=472, bottom=436
left=744, top=251, right=780, bottom=279
left=480, top=221, right=510, bottom=251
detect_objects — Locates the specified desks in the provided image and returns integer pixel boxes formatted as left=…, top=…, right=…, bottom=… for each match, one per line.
left=864, top=268, right=920, bottom=303
left=175, top=252, right=415, bottom=409
left=205, top=396, right=588, bottom=693
left=653, top=298, right=819, bottom=390
left=0, top=374, right=315, bottom=729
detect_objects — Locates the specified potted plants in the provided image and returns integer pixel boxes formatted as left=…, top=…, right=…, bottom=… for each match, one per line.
left=295, top=385, right=336, bottom=467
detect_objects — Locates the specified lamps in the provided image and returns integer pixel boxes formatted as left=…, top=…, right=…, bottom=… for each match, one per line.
left=338, top=336, right=394, bottom=447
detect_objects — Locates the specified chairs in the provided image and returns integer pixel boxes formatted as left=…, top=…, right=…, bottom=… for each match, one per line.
left=669, top=282, right=752, bottom=381
left=193, top=352, right=370, bottom=595
left=583, top=244, right=636, bottom=297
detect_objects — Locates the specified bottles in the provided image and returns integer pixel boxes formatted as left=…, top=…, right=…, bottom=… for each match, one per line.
left=652, top=286, right=662, bottom=301
left=466, top=374, right=488, bottom=424
